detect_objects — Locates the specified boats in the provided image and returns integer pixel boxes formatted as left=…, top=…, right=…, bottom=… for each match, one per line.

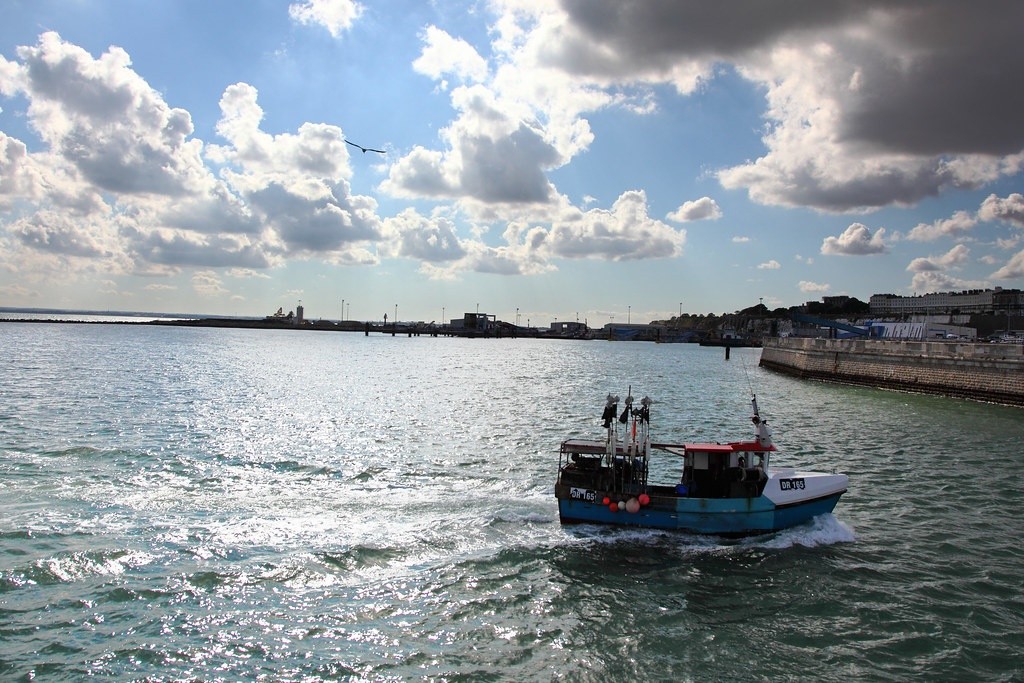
left=554, top=385, right=849, bottom=535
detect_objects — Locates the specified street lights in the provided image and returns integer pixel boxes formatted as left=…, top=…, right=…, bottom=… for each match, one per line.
left=443, top=307, right=445, bottom=324
left=628, top=305, right=631, bottom=324
left=759, top=297, right=763, bottom=304
left=515, top=307, right=522, bottom=326
left=395, top=304, right=398, bottom=323
left=679, top=302, right=682, bottom=316
left=346, top=303, right=349, bottom=321
left=342, top=299, right=345, bottom=321
left=555, top=318, right=557, bottom=322
left=527, top=319, right=529, bottom=327
left=477, top=303, right=479, bottom=312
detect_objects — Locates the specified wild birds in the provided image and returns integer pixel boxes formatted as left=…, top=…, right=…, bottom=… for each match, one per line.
left=345, top=139, right=386, bottom=154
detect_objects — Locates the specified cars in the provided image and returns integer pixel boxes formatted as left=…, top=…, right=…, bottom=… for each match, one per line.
left=978, top=336, right=1000, bottom=343
